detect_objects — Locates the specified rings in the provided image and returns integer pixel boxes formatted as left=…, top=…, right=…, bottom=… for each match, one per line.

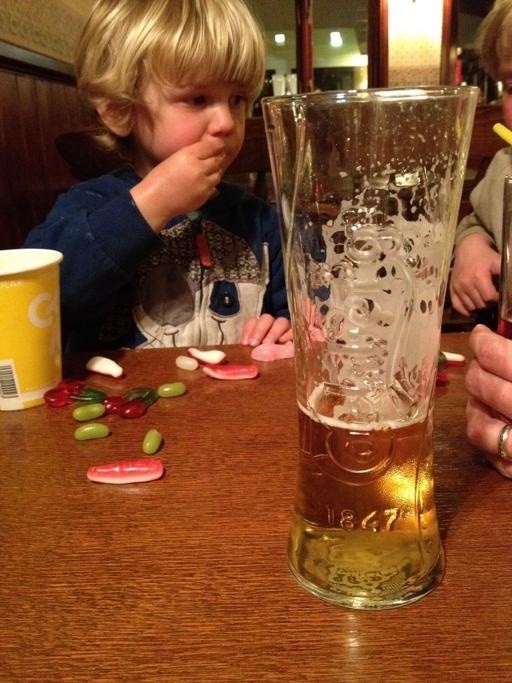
left=496, top=421, right=512, bottom=464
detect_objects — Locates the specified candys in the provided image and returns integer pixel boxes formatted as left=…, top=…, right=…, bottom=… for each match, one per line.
left=44, top=341, right=294, bottom=441
left=436, top=350, right=466, bottom=386
left=142, top=429, right=162, bottom=454
left=86, top=458, right=165, bottom=485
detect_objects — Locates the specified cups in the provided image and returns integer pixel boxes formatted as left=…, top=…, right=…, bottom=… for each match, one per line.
left=0, top=246, right=65, bottom=413
left=259, top=83, right=481, bottom=611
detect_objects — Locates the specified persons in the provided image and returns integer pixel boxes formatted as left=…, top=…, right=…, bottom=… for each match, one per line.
left=461, top=320, right=512, bottom=483
left=23, top=0, right=338, bottom=354
left=448, top=0, right=512, bottom=315
